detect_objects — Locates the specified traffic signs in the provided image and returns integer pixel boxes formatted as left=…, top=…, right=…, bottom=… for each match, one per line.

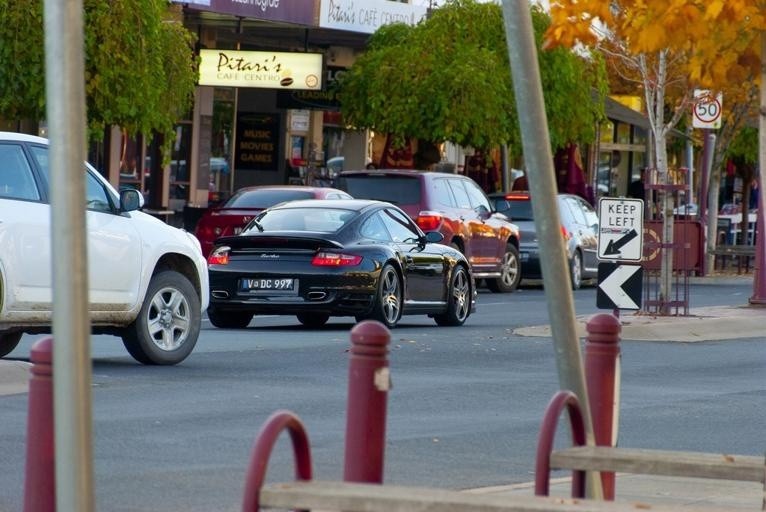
left=594, top=195, right=645, bottom=264
left=688, top=87, right=728, bottom=129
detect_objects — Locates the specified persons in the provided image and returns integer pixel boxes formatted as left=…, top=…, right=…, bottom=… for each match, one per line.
left=625, top=169, right=664, bottom=220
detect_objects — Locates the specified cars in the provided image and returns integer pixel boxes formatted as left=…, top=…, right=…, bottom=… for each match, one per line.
left=653, top=202, right=759, bottom=224
left=598, top=160, right=638, bottom=194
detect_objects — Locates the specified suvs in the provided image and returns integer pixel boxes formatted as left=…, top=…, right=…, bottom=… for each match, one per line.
left=0, top=132, right=213, bottom=362
